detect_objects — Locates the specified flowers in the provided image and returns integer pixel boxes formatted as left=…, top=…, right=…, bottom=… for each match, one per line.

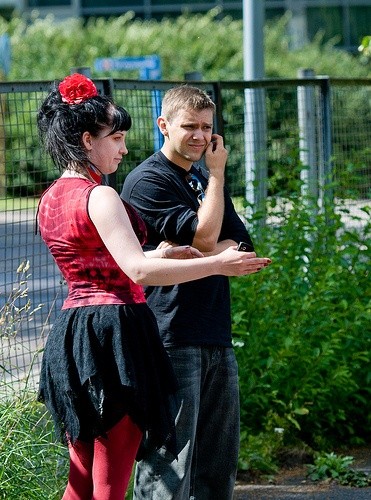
left=58, top=72, right=100, bottom=106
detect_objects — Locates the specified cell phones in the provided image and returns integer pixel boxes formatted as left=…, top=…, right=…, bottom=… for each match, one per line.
left=237, top=241, right=254, bottom=252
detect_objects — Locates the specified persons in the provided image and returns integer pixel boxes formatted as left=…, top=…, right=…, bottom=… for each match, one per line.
left=117, top=85, right=253, bottom=500
left=34, top=72, right=272, bottom=500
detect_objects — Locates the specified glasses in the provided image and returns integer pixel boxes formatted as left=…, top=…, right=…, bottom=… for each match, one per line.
left=185, top=170, right=205, bottom=204
